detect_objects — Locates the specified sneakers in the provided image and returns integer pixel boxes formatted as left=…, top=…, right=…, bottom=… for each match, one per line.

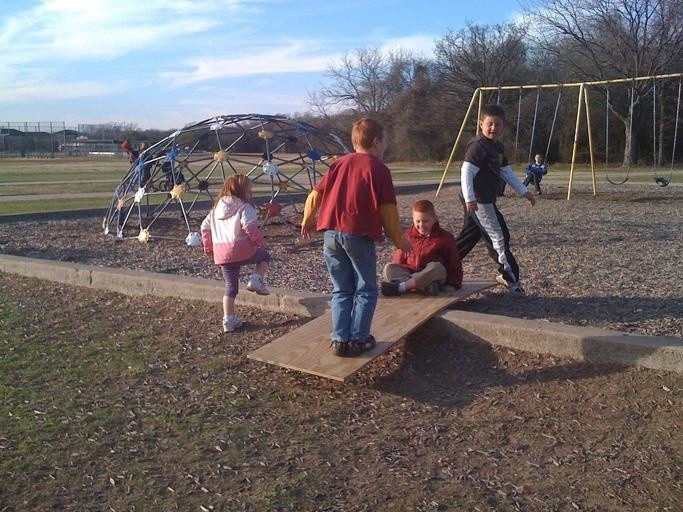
left=427, top=280, right=439, bottom=296
left=496, top=271, right=526, bottom=297
left=328, top=334, right=376, bottom=357
left=223, top=315, right=244, bottom=333
left=246, top=274, right=270, bottom=295
left=536, top=191, right=542, bottom=196
left=381, top=280, right=405, bottom=297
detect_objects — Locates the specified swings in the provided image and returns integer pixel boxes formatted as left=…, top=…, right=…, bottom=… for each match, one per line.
left=525, top=89, right=562, bottom=184
left=652, top=81, right=681, bottom=187
left=605, top=87, right=633, bottom=185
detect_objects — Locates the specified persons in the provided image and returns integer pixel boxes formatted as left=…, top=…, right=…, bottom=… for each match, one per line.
left=301, top=118, right=413, bottom=357
left=522, top=154, right=547, bottom=195
left=381, top=200, right=463, bottom=296
left=201, top=174, right=270, bottom=333
left=128, top=142, right=158, bottom=192
left=455, top=105, right=535, bottom=298
